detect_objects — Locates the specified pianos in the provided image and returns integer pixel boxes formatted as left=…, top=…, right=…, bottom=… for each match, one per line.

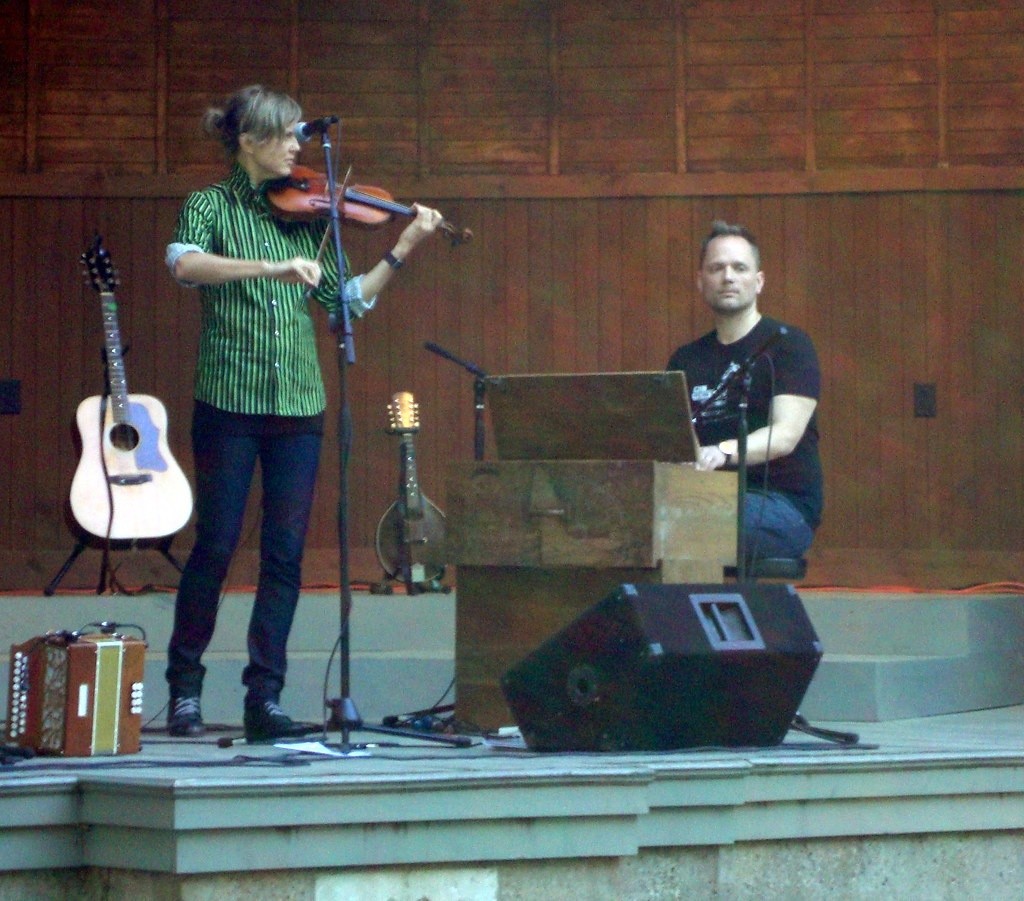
left=455, top=369, right=733, bottom=729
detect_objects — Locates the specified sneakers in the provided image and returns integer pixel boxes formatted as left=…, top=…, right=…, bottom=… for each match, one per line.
left=243, top=698, right=305, bottom=743
left=168, top=695, right=206, bottom=738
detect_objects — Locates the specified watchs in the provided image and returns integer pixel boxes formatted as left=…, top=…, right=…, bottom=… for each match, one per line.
left=716, top=439, right=736, bottom=471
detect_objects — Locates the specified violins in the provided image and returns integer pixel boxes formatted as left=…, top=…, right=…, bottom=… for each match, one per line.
left=266, top=163, right=475, bottom=248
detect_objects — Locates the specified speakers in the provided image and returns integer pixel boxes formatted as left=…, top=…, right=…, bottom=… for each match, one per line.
left=508, top=580, right=816, bottom=755
left=7, top=632, right=148, bottom=758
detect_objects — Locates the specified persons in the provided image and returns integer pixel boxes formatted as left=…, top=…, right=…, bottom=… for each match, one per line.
left=159, top=82, right=443, bottom=740
left=665, top=218, right=824, bottom=563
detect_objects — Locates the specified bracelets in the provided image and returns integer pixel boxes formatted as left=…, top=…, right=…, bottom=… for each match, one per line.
left=383, top=251, right=403, bottom=269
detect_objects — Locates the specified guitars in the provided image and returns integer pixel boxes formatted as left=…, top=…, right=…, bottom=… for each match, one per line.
left=70, top=240, right=195, bottom=540
left=376, top=389, right=451, bottom=584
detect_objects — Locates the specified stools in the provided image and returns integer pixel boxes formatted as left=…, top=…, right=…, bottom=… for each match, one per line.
left=731, top=556, right=865, bottom=745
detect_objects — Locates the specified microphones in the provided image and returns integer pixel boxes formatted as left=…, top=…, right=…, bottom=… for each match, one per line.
left=292, top=116, right=339, bottom=141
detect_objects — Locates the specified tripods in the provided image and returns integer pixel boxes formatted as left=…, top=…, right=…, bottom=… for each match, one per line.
left=215, top=142, right=471, bottom=750
